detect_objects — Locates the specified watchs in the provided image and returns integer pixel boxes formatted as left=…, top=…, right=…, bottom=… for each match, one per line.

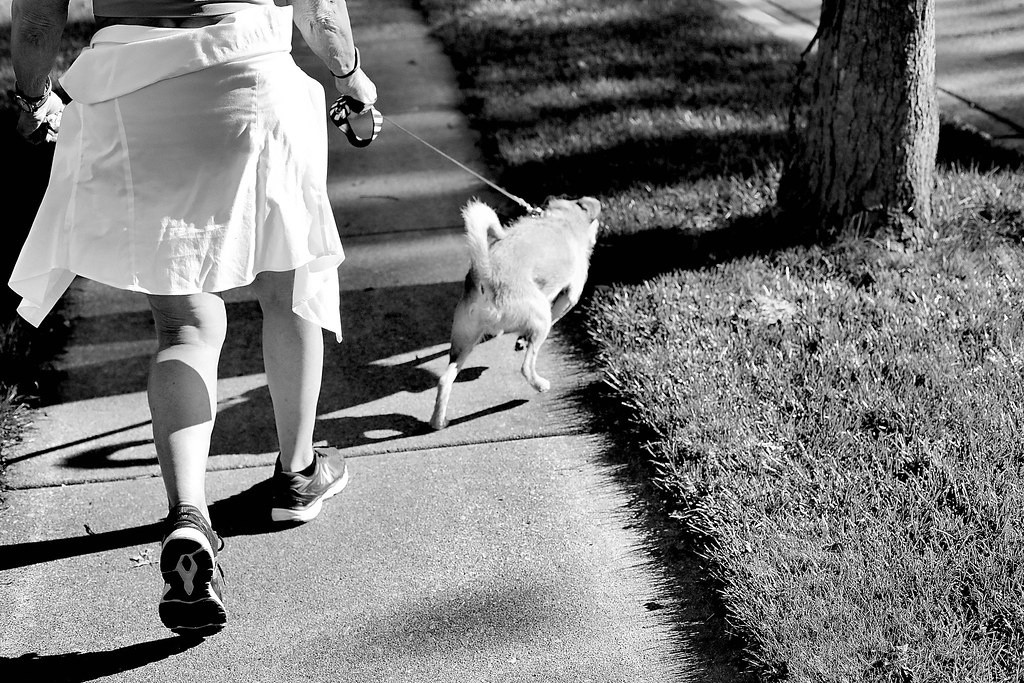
left=14, top=75, right=53, bottom=113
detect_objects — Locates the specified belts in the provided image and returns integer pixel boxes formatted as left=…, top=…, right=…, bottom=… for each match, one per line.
left=95, top=12, right=237, bottom=31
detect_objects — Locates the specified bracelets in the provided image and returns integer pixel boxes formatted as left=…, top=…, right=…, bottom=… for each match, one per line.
left=330, top=47, right=361, bottom=78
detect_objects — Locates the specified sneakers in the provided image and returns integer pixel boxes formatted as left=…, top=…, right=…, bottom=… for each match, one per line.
left=270, top=447, right=348, bottom=523
left=159, top=505, right=228, bottom=637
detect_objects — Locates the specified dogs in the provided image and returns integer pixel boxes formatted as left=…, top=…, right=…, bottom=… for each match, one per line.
left=430, top=194, right=602, bottom=431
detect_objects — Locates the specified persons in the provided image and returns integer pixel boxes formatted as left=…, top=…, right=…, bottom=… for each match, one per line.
left=11, top=0, right=378, bottom=637
